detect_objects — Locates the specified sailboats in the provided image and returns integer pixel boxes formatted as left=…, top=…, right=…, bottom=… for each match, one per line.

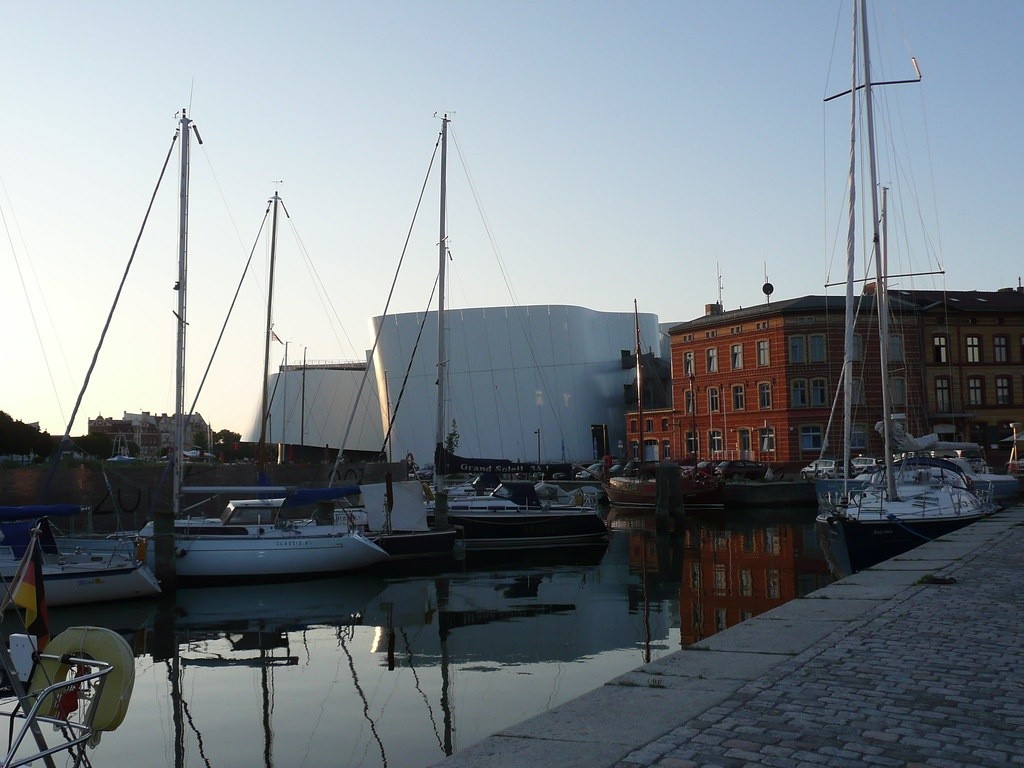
left=52, top=106, right=392, bottom=582
left=254, top=1, right=1024, bottom=563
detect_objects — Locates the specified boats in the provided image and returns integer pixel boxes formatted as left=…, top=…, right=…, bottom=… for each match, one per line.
left=1, top=457, right=163, bottom=611
